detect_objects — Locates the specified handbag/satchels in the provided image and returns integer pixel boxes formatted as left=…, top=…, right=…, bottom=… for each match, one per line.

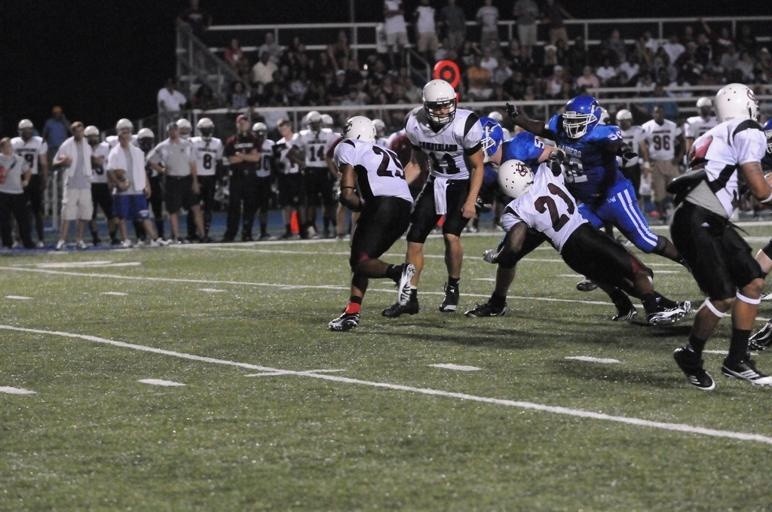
left=0, top=154, right=17, bottom=184
left=638, top=172, right=652, bottom=196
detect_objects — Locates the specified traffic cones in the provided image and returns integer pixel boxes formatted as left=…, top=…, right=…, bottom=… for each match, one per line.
left=291, top=210, right=301, bottom=235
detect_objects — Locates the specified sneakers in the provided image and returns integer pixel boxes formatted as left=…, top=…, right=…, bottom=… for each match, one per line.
left=328, top=313, right=359, bottom=330
left=611, top=299, right=638, bottom=321
left=747, top=321, right=772, bottom=351
left=640, top=289, right=691, bottom=327
left=391, top=262, right=416, bottom=307
left=647, top=206, right=676, bottom=224
left=673, top=346, right=716, bottom=391
left=381, top=289, right=419, bottom=317
left=2, top=227, right=347, bottom=252
left=437, top=283, right=460, bottom=311
left=575, top=279, right=598, bottom=291
left=721, top=356, right=772, bottom=388
left=462, top=298, right=512, bottom=319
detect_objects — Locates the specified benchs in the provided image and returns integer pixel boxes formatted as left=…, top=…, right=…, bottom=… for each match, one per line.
left=152, top=0, right=772, bottom=234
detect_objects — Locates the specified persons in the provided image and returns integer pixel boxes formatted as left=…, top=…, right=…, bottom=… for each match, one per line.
left=325, top=81, right=770, bottom=391
left=1, top=2, right=771, bottom=253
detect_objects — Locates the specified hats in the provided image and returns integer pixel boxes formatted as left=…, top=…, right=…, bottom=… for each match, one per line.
left=277, top=118, right=289, bottom=127
left=236, top=114, right=249, bottom=122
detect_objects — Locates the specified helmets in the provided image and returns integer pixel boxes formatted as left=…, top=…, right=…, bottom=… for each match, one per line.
left=713, top=81, right=759, bottom=123
left=561, top=94, right=603, bottom=140
left=303, top=110, right=334, bottom=125
left=252, top=121, right=268, bottom=131
left=422, top=79, right=457, bottom=132
left=17, top=105, right=216, bottom=141
left=498, top=158, right=535, bottom=199
left=342, top=115, right=377, bottom=146
left=616, top=109, right=633, bottom=121
left=696, top=96, right=713, bottom=108
left=487, top=111, right=503, bottom=124
left=371, top=118, right=385, bottom=133
